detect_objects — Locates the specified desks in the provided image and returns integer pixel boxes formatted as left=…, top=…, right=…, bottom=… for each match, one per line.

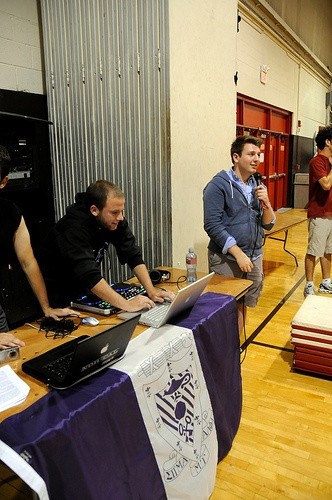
left=262, top=212, right=308, bottom=267
left=0, top=266, right=254, bottom=500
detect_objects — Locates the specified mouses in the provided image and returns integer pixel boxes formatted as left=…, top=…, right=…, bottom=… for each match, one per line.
left=81, top=316, right=99, bottom=326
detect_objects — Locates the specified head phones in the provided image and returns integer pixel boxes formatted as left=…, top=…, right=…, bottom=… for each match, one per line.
left=148, top=269, right=171, bottom=285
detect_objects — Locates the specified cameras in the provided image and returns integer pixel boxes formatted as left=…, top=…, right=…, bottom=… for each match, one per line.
left=0, top=346, right=21, bottom=363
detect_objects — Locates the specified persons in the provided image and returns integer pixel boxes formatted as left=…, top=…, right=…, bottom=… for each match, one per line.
left=30, top=180, right=175, bottom=312
left=303, top=125, right=332, bottom=297
left=202, top=136, right=276, bottom=336
left=0, top=144, right=80, bottom=332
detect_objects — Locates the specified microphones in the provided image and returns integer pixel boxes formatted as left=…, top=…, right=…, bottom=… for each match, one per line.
left=254, top=172, right=266, bottom=209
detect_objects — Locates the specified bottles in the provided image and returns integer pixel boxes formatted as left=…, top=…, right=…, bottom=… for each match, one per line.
left=186, top=249, right=197, bottom=281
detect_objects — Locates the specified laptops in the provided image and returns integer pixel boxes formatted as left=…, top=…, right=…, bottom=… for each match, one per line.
left=117, top=272, right=215, bottom=329
left=22, top=313, right=142, bottom=389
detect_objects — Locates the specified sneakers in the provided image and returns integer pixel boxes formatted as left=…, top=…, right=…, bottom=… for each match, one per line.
left=318, top=282, right=332, bottom=295
left=304, top=284, right=316, bottom=298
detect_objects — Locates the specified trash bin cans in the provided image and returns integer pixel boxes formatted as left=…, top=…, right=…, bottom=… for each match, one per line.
left=294, top=173, right=309, bottom=209
